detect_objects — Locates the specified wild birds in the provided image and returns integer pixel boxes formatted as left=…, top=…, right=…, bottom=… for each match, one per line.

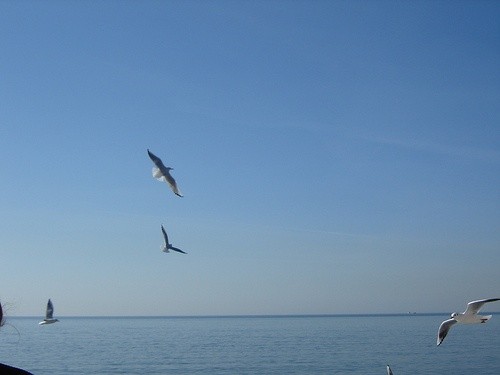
left=161, top=224, right=187, bottom=254
left=38, top=299, right=59, bottom=325
left=437, top=298, right=500, bottom=347
left=147, top=150, right=184, bottom=198
